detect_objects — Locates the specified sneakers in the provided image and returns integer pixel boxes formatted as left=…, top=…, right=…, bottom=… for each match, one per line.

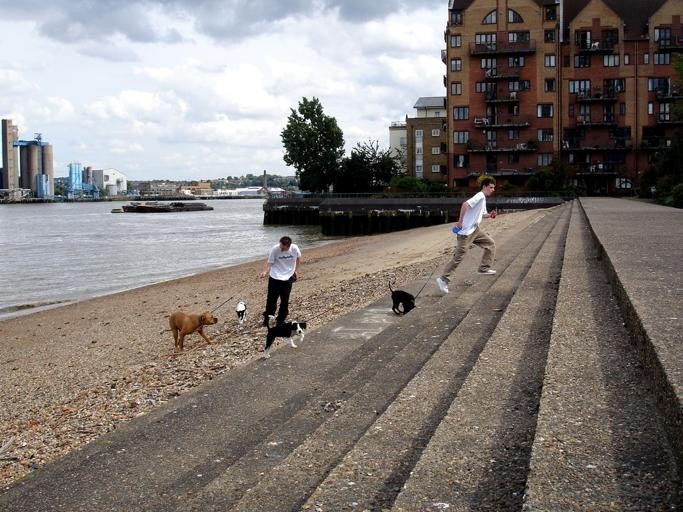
left=436, top=278, right=449, bottom=293
left=477, top=269, right=496, bottom=275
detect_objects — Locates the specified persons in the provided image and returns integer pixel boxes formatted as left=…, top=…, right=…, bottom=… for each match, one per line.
left=436, top=173, right=496, bottom=293
left=258, top=236, right=302, bottom=328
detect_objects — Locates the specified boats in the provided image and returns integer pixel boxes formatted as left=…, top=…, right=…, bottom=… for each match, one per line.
left=122, top=201, right=215, bottom=212
left=109, top=209, right=123, bottom=214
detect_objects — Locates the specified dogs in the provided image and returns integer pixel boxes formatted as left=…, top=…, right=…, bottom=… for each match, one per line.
left=235, top=300, right=247, bottom=324
left=261, top=312, right=307, bottom=360
left=159, top=310, right=219, bottom=351
left=388, top=280, right=416, bottom=316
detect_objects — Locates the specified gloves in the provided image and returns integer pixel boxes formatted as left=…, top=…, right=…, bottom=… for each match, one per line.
left=289, top=273, right=297, bottom=283
